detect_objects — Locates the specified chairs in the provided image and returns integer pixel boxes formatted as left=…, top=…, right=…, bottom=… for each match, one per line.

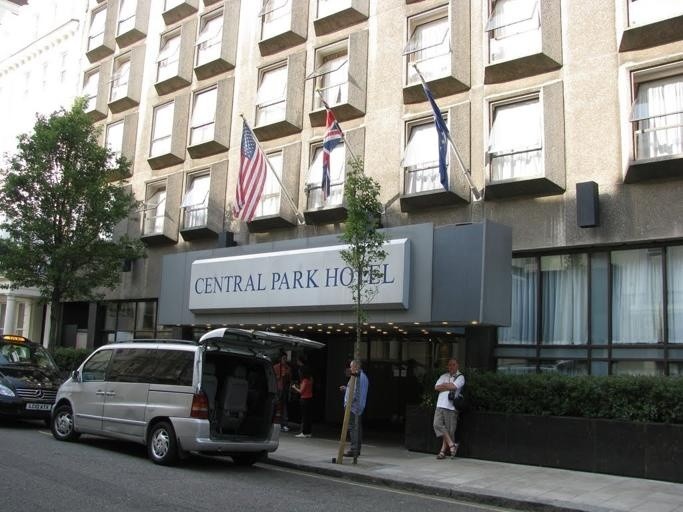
left=202, top=362, right=249, bottom=434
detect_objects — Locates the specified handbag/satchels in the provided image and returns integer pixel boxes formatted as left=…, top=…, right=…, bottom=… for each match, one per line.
left=453, top=397, right=464, bottom=410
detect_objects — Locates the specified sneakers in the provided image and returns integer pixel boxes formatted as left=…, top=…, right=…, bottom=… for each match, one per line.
left=282, top=426, right=289, bottom=432
left=295, top=431, right=312, bottom=438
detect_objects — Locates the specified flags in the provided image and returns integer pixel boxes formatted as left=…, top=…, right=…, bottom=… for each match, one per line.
left=317, top=99, right=343, bottom=204
left=229, top=120, right=268, bottom=227
left=416, top=76, right=453, bottom=196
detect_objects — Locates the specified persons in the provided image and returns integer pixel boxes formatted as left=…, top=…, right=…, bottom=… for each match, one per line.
left=295, top=353, right=314, bottom=380
left=272, top=352, right=290, bottom=434
left=289, top=366, right=313, bottom=440
left=431, top=358, right=466, bottom=460
left=338, top=359, right=370, bottom=457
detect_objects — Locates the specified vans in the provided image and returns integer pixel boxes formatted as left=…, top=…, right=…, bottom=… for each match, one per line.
left=51, top=328, right=326, bottom=465
left=0, top=335, right=65, bottom=419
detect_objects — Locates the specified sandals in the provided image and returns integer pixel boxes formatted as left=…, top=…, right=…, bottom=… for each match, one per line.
left=436, top=449, right=447, bottom=460
left=449, top=442, right=459, bottom=459
